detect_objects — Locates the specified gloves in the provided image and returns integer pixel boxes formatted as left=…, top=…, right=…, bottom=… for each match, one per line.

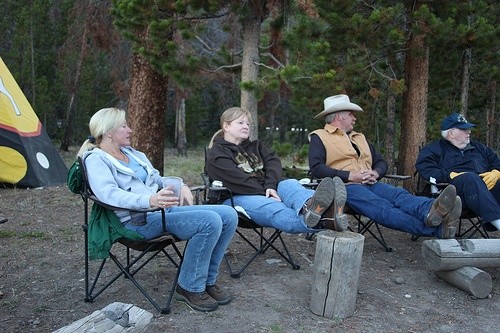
left=478, top=169, right=500, bottom=190
left=451, top=172, right=468, bottom=178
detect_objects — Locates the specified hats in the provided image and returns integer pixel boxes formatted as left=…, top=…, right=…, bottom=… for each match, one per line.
left=440, top=113, right=476, bottom=130
left=313, top=94, right=365, bottom=118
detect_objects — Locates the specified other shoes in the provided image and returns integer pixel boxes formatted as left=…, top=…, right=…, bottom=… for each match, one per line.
left=426, top=185, right=456, bottom=227
left=438, top=195, right=462, bottom=238
left=303, top=177, right=335, bottom=228
left=320, top=177, right=348, bottom=231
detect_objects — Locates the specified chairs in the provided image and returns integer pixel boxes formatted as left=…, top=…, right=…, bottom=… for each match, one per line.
left=67, top=155, right=204, bottom=314
left=193, top=146, right=302, bottom=279
left=413, top=141, right=490, bottom=242
left=302, top=170, right=411, bottom=253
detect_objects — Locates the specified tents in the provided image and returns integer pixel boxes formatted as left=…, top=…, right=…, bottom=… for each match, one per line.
left=0, top=56, right=70, bottom=187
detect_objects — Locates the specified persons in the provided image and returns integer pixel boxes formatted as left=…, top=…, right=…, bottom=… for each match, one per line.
left=415, top=113, right=500, bottom=231
left=307, top=94, right=462, bottom=238
left=206, top=107, right=348, bottom=233
left=77, top=106, right=239, bottom=311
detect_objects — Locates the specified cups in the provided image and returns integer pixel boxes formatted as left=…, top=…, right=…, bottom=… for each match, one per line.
left=210, top=180, right=223, bottom=202
left=161, top=176, right=182, bottom=199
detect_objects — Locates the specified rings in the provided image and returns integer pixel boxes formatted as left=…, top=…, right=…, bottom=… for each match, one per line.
left=162, top=201, right=166, bottom=206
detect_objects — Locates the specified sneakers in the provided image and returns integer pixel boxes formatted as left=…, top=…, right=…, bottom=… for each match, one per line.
left=174, top=283, right=218, bottom=311
left=205, top=285, right=230, bottom=305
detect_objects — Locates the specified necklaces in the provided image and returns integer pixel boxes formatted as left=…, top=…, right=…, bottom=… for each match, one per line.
left=119, top=151, right=126, bottom=161
